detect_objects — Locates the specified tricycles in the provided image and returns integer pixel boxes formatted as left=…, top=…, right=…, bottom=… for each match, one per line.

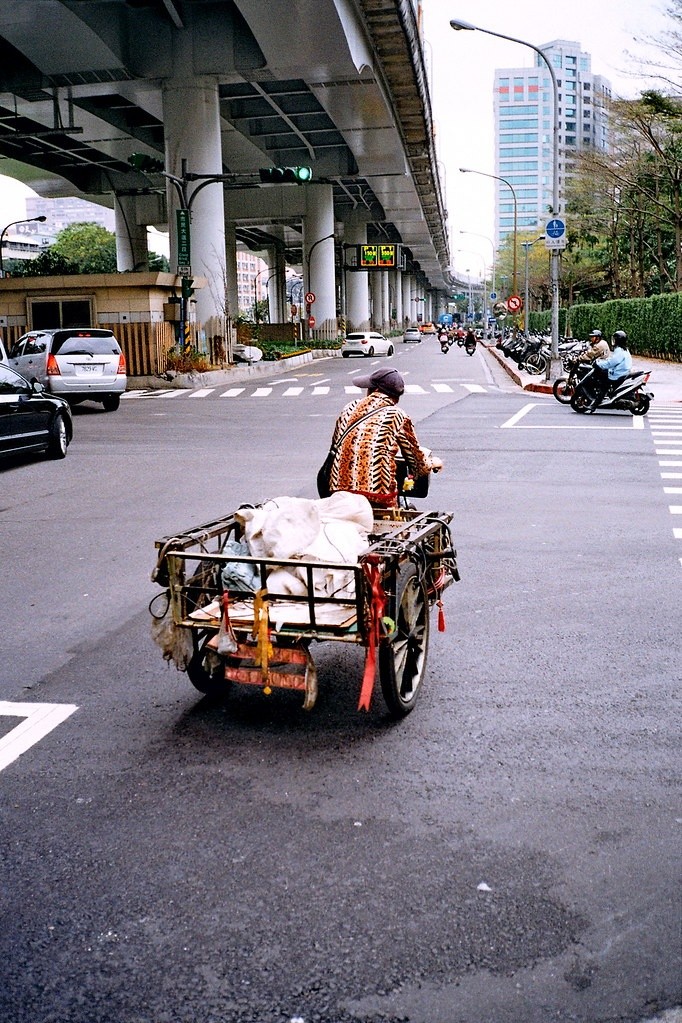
left=154, top=444, right=454, bottom=719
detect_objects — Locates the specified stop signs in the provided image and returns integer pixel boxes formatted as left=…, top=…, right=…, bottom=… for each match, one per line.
left=309, top=317, right=315, bottom=327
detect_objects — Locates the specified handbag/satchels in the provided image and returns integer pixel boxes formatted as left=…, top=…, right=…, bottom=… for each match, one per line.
left=317, top=452, right=332, bottom=499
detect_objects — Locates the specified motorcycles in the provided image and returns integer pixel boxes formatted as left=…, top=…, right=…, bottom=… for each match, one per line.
left=436, top=323, right=592, bottom=374
left=553, top=347, right=614, bottom=404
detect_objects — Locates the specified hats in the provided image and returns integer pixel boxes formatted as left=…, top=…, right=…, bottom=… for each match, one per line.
left=353, top=368, right=404, bottom=395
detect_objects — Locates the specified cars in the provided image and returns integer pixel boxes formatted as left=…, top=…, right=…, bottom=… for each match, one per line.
left=341, top=331, right=395, bottom=358
left=0, top=363, right=73, bottom=469
left=0, top=339, right=10, bottom=366
left=421, top=323, right=436, bottom=335
left=403, top=328, right=422, bottom=343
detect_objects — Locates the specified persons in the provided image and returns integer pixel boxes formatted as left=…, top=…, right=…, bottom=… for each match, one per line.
left=329, top=367, right=443, bottom=510
left=583, top=331, right=632, bottom=414
left=436, top=324, right=476, bottom=346
left=577, top=330, right=611, bottom=362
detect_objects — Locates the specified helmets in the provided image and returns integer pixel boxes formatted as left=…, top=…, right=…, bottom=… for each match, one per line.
left=589, top=330, right=602, bottom=336
left=611, top=330, right=626, bottom=345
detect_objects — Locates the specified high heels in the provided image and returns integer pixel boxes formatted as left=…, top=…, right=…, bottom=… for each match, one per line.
left=583, top=404, right=596, bottom=414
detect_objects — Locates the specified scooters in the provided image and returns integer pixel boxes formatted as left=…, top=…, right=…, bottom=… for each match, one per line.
left=570, top=357, right=654, bottom=416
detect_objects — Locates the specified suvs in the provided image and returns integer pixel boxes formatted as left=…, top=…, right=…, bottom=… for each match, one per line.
left=8, top=325, right=127, bottom=411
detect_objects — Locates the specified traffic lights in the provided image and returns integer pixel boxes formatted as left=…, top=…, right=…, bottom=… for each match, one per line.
left=259, top=168, right=313, bottom=183
left=420, top=298, right=426, bottom=301
left=132, top=152, right=165, bottom=174
left=451, top=295, right=458, bottom=299
left=181, top=279, right=194, bottom=298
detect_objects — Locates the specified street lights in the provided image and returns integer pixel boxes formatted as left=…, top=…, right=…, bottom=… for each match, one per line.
left=450, top=18, right=559, bottom=377
left=458, top=168, right=545, bottom=335
left=0, top=216, right=47, bottom=271
left=255, top=233, right=337, bottom=337
